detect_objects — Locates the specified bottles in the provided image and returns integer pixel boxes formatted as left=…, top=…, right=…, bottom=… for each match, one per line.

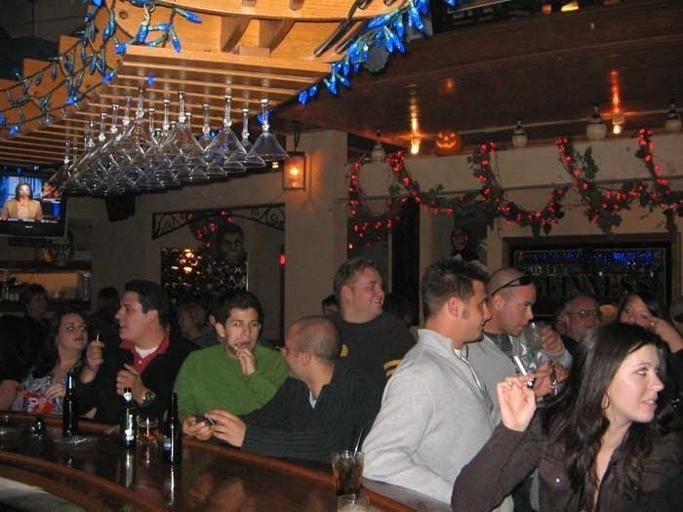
left=162, top=392, right=182, bottom=468
left=62, top=369, right=78, bottom=440
left=120, top=387, right=137, bottom=449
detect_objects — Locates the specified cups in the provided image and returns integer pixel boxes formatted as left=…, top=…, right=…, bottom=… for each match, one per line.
left=523, top=323, right=544, bottom=351
left=59, top=90, right=159, bottom=192
left=134, top=413, right=161, bottom=445
left=337, top=494, right=370, bottom=512
left=203, top=96, right=247, bottom=154
left=159, top=91, right=205, bottom=156
left=159, top=156, right=286, bottom=188
left=331, top=455, right=364, bottom=494
left=245, top=99, right=289, bottom=162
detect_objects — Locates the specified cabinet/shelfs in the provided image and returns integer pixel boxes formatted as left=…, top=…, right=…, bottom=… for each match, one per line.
left=501, top=234, right=679, bottom=323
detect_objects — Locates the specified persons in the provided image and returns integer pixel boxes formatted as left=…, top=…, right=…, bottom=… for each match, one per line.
left=86, top=288, right=125, bottom=344
left=14, top=310, right=100, bottom=419
left=61, top=281, right=204, bottom=431
left=207, top=316, right=385, bottom=467
left=453, top=267, right=572, bottom=430
left=1, top=316, right=50, bottom=413
left=555, top=297, right=601, bottom=353
left=332, top=263, right=418, bottom=414
left=447, top=230, right=478, bottom=263
left=171, top=291, right=288, bottom=441
left=619, top=294, right=683, bottom=379
left=15, top=283, right=60, bottom=342
left=1, top=181, right=43, bottom=221
left=382, top=290, right=419, bottom=344
left=323, top=296, right=337, bottom=318
left=361, top=262, right=517, bottom=510
left=451, top=321, right=683, bottom=511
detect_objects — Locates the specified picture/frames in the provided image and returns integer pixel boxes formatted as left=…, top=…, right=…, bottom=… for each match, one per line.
left=152, top=204, right=285, bottom=349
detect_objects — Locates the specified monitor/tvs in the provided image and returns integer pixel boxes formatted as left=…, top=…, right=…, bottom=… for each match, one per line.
left=0, top=163, right=69, bottom=242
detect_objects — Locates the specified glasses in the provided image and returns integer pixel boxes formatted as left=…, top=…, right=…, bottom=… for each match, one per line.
left=557, top=308, right=604, bottom=323
left=225, top=319, right=263, bottom=329
left=490, top=274, right=540, bottom=296
left=344, top=255, right=378, bottom=269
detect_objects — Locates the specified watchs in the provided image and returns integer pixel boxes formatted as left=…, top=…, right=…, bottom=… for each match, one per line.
left=142, top=387, right=153, bottom=407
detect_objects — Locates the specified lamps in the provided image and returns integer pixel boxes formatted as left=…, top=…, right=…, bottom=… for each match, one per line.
left=433, top=127, right=462, bottom=156
left=282, top=151, right=305, bottom=190
